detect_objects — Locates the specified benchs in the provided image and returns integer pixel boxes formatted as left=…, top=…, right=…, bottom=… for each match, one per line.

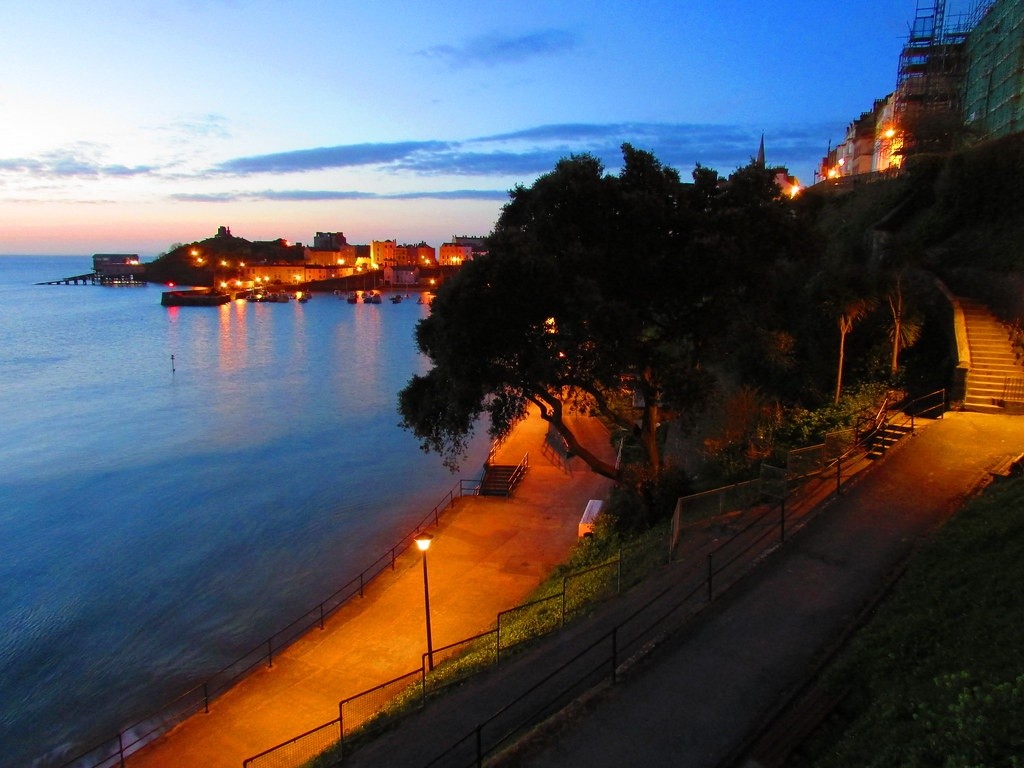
left=578, top=499, right=603, bottom=537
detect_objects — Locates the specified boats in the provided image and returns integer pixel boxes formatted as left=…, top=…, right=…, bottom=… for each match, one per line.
left=247, top=284, right=438, bottom=306
